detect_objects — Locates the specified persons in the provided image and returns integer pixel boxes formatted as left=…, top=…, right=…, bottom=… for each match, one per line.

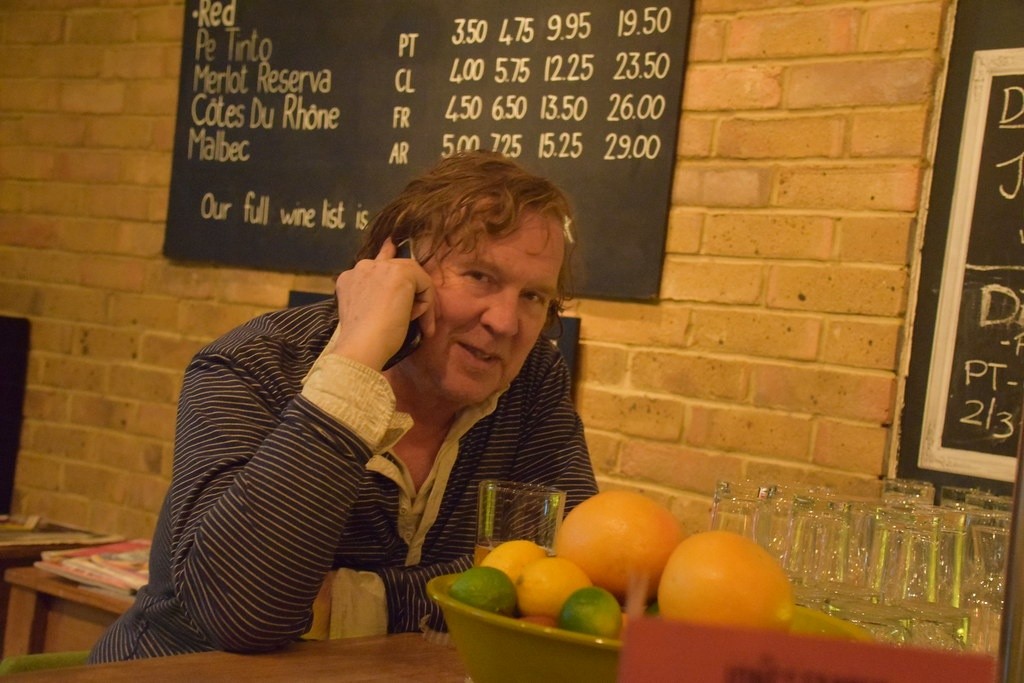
left=88, top=148, right=599, bottom=665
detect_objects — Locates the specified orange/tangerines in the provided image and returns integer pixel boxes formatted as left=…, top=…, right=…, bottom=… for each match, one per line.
left=446, top=540, right=661, bottom=642
left=553, top=491, right=794, bottom=629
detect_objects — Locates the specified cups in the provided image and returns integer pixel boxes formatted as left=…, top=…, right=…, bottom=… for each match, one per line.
left=710, top=472, right=1012, bottom=655
left=474, top=479, right=566, bottom=571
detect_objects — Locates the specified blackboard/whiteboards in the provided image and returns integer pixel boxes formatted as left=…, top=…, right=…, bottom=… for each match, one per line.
left=163, top=0, right=694, bottom=303
left=880, top=0, right=1024, bottom=499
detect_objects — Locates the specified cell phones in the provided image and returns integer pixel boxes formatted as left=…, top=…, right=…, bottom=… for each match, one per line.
left=382, top=237, right=423, bottom=373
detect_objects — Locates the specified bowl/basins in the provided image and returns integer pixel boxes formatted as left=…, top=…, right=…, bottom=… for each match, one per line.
left=426, top=572, right=622, bottom=683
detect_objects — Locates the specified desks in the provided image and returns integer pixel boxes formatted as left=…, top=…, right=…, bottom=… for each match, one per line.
left=0, top=624, right=477, bottom=683
left=0, top=516, right=153, bottom=657
